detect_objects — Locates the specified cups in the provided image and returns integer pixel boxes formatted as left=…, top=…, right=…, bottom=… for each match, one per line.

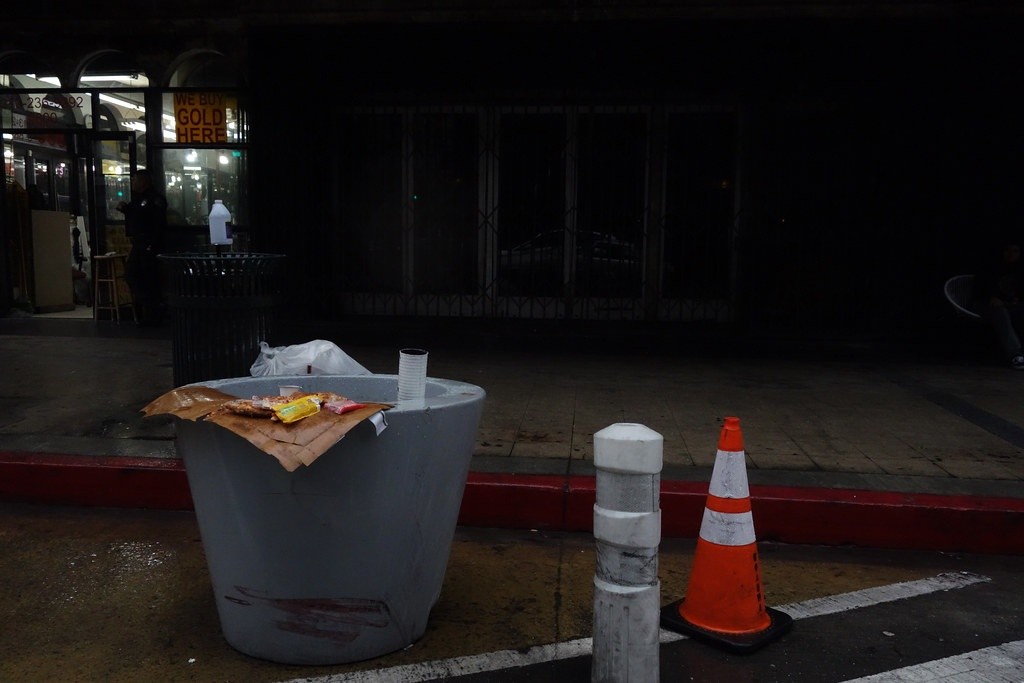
left=278, top=385, right=300, bottom=396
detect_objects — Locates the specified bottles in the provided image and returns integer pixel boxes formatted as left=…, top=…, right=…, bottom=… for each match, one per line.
left=208, top=199, right=234, bottom=245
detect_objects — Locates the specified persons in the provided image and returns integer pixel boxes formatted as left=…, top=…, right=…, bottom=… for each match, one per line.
left=972, top=237, right=1024, bottom=370
left=116, top=170, right=168, bottom=327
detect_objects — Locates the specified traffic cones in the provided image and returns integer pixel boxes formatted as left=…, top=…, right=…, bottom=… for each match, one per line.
left=660, top=416, right=797, bottom=652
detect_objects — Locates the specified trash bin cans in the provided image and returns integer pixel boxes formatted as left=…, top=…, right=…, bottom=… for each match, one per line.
left=175, top=373, right=488, bottom=667
left=157, top=252, right=288, bottom=391
